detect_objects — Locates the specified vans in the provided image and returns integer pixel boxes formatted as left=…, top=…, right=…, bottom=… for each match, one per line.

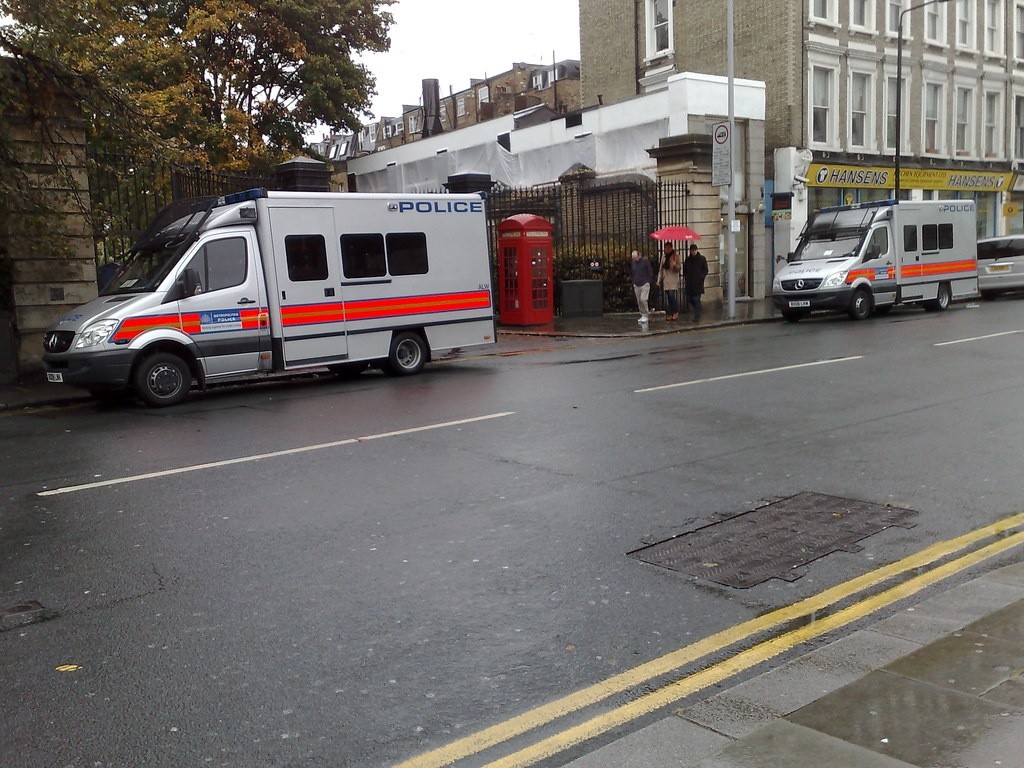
left=978, top=233, right=1024, bottom=297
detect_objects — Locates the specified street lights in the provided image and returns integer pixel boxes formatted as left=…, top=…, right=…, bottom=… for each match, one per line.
left=894, top=0, right=951, bottom=203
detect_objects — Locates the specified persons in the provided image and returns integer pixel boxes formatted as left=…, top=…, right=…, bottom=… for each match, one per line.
left=682, top=244, right=709, bottom=323
left=657, top=241, right=682, bottom=321
left=630, top=250, right=653, bottom=322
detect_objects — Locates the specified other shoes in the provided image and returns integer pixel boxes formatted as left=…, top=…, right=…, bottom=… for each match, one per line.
left=666, top=312, right=678, bottom=320
left=693, top=317, right=699, bottom=321
left=638, top=316, right=648, bottom=322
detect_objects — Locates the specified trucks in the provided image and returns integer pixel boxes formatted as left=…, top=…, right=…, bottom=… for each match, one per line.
left=46, top=188, right=499, bottom=409
left=773, top=197, right=980, bottom=319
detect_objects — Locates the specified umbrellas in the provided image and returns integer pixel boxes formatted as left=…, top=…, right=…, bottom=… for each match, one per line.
left=649, top=226, right=703, bottom=240
